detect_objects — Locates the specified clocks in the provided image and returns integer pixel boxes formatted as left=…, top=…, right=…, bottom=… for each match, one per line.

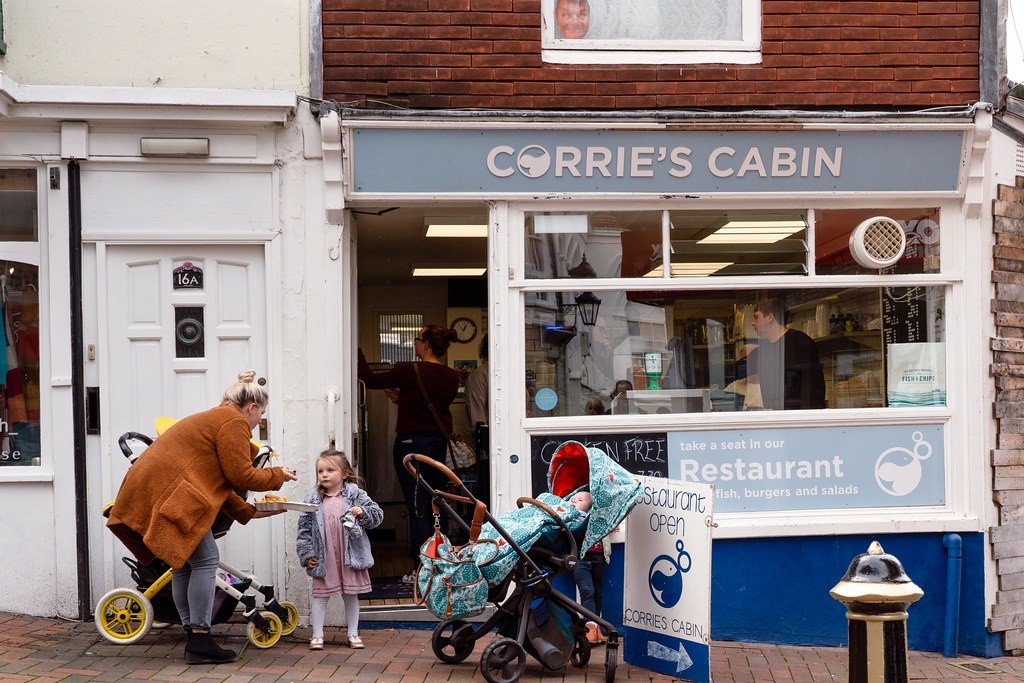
left=450, top=317, right=477, bottom=344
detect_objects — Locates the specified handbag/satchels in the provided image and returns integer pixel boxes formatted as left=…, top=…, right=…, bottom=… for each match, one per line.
left=412, top=489, right=499, bottom=622
left=446, top=431, right=477, bottom=476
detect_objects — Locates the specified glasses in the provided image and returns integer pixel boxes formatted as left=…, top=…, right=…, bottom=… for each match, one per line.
left=415, top=338, right=425, bottom=342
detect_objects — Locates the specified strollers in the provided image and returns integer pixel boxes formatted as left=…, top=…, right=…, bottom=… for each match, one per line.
left=404, top=440, right=646, bottom=683
left=95, top=430, right=308, bottom=649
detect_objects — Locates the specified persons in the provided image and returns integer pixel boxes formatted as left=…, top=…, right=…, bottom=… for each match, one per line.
left=471, top=492, right=594, bottom=562
left=105, top=369, right=298, bottom=666
left=464, top=333, right=491, bottom=527
left=585, top=397, right=606, bottom=416
left=297, top=450, right=384, bottom=651
left=578, top=307, right=617, bottom=417
left=355, top=326, right=464, bottom=586
left=555, top=1, right=591, bottom=40
left=573, top=526, right=622, bottom=646
left=605, top=381, right=649, bottom=416
left=684, top=296, right=830, bottom=411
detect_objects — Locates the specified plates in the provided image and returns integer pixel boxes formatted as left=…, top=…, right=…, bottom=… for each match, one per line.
left=254, top=502, right=319, bottom=513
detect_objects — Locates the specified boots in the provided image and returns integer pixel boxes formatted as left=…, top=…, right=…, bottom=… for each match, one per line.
left=184, top=625, right=240, bottom=664
left=585, top=621, right=598, bottom=643
left=598, top=625, right=608, bottom=642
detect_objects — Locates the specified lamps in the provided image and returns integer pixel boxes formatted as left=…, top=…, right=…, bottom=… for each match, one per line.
left=422, top=216, right=489, bottom=237
left=641, top=254, right=736, bottom=277
left=557, top=291, right=601, bottom=326
left=692, top=215, right=807, bottom=244
left=411, top=263, right=488, bottom=277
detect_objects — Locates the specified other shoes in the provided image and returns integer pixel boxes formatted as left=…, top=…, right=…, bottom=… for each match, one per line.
left=348, top=636, right=365, bottom=648
left=308, top=636, right=327, bottom=650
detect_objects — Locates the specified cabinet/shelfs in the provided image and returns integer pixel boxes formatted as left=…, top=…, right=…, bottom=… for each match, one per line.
left=675, top=314, right=734, bottom=389
